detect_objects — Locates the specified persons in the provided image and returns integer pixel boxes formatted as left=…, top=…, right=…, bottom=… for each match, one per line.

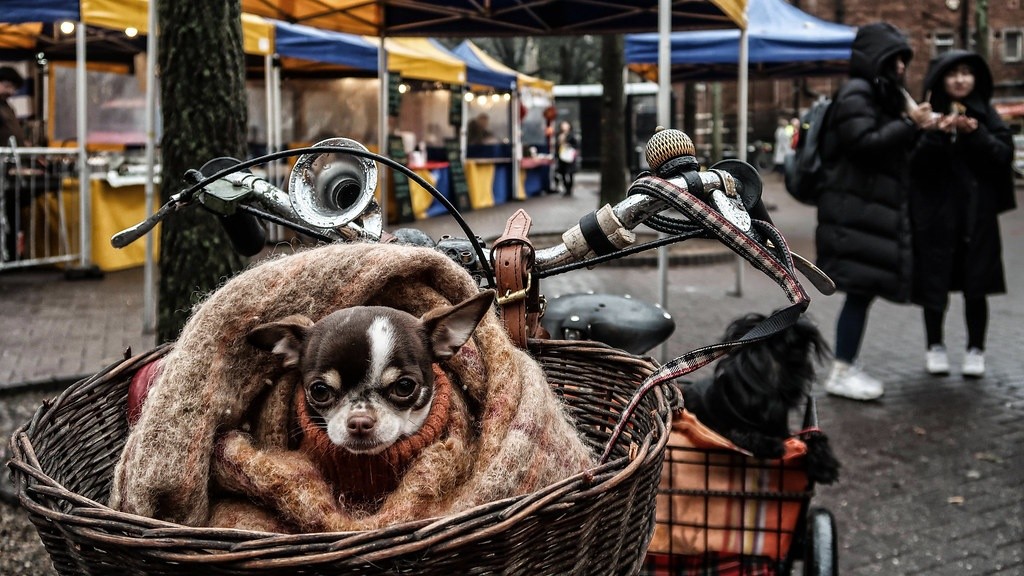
left=0, top=66, right=32, bottom=262
left=814, top=23, right=939, bottom=400
left=772, top=118, right=801, bottom=175
left=910, top=50, right=1017, bottom=376
left=467, top=113, right=497, bottom=144
left=555, top=120, right=579, bottom=197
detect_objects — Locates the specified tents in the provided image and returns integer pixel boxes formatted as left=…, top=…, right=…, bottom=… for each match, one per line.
left=0, top=0, right=747, bottom=271
left=621, top=0, right=857, bottom=166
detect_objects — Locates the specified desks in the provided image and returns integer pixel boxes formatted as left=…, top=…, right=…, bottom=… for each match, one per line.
left=21, top=158, right=554, bottom=272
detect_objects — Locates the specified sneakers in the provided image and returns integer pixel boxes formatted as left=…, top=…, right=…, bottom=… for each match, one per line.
left=824, top=363, right=887, bottom=405
left=962, top=348, right=987, bottom=379
left=925, top=347, right=952, bottom=377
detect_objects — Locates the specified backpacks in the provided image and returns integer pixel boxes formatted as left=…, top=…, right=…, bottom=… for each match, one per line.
left=782, top=92, right=832, bottom=203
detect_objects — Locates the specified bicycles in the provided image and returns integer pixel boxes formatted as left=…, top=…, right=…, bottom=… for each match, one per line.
left=5, top=125, right=838, bottom=575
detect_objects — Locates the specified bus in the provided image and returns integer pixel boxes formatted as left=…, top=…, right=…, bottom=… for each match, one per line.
left=551, top=83, right=660, bottom=170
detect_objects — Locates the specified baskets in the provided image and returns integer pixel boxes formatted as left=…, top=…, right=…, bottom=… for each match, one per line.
left=656, top=389, right=822, bottom=572
left=8, top=331, right=686, bottom=571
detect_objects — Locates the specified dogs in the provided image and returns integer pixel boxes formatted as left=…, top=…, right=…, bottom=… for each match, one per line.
left=682, top=309, right=834, bottom=450
left=250, top=290, right=498, bottom=454
